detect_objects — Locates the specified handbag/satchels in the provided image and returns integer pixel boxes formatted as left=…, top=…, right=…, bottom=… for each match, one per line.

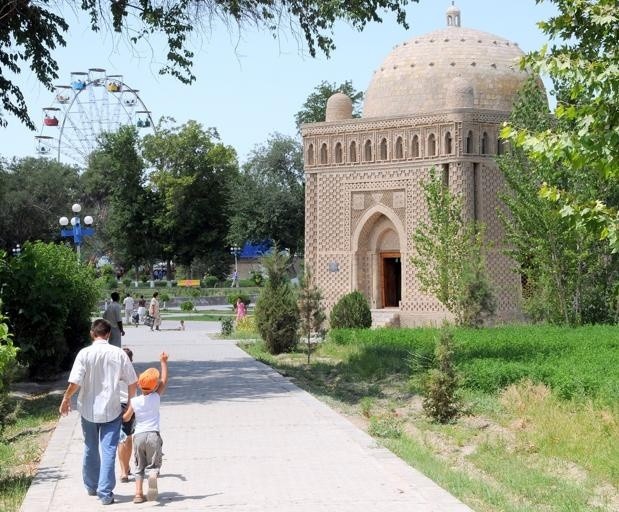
left=144, top=315, right=155, bottom=327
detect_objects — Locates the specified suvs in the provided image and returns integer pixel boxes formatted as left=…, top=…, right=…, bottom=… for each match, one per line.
left=143, top=260, right=177, bottom=280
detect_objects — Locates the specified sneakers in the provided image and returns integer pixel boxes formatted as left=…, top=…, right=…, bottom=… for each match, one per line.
left=88, top=491, right=114, bottom=505
left=133, top=493, right=158, bottom=503
left=119, top=466, right=131, bottom=483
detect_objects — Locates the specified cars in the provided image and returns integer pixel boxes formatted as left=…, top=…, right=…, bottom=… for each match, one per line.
left=95, top=254, right=123, bottom=280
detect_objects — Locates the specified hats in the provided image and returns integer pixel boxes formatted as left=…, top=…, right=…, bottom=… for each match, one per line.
left=139, top=368, right=160, bottom=393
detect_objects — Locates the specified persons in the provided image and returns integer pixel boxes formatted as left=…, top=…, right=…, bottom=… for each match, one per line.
left=103, top=292, right=125, bottom=349
left=235, top=297, right=247, bottom=323
left=230, top=268, right=240, bottom=288
left=153, top=269, right=164, bottom=280
left=123, top=292, right=186, bottom=331
left=59, top=319, right=169, bottom=505
left=280, top=255, right=300, bottom=288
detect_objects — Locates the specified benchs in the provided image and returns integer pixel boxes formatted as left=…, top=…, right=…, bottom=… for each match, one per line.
left=176, top=279, right=201, bottom=287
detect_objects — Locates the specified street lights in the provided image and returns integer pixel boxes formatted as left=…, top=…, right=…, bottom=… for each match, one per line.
left=58, top=201, right=96, bottom=269
left=229, top=242, right=242, bottom=272
left=11, top=243, right=22, bottom=257
